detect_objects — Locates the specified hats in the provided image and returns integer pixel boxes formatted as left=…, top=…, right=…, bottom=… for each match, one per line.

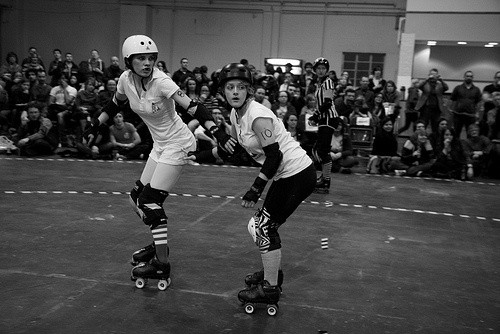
left=285, top=63, right=293, bottom=67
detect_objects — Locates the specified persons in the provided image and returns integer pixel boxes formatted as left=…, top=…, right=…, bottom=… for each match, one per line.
left=308, top=56, right=339, bottom=194
left=83, top=33, right=238, bottom=292
left=0, top=47, right=500, bottom=188
left=181, top=62, right=318, bottom=317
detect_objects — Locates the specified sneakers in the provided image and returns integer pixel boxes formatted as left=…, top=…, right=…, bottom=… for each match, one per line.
left=316, top=177, right=330, bottom=188
left=317, top=174, right=323, bottom=182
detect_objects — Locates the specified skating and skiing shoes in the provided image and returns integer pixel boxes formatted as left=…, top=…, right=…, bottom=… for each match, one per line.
left=238, top=279, right=281, bottom=316
left=133, top=241, right=169, bottom=261
left=245, top=270, right=283, bottom=294
left=131, top=255, right=171, bottom=290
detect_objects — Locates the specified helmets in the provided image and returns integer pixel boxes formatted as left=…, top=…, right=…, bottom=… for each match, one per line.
left=313, top=57, right=329, bottom=70
left=122, top=34, right=158, bottom=68
left=219, top=63, right=253, bottom=85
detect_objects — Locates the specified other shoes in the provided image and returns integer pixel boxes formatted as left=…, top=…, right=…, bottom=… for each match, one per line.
left=115, top=157, right=124, bottom=162
left=416, top=171, right=422, bottom=177
left=394, top=170, right=406, bottom=176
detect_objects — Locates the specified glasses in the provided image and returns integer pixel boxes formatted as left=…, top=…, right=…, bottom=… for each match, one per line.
left=195, top=72, right=200, bottom=74
left=290, top=89, right=296, bottom=92
